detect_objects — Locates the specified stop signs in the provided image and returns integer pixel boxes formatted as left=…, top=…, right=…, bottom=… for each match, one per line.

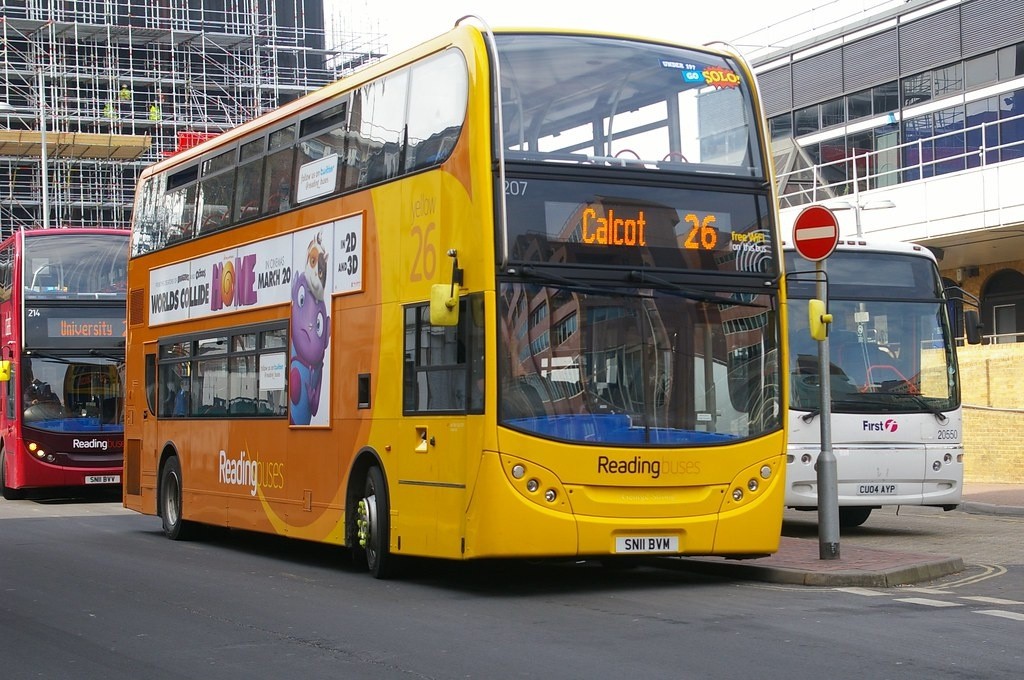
left=792, top=205, right=840, bottom=262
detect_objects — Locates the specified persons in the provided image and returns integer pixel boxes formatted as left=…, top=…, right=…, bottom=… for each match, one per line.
left=103, top=101, right=117, bottom=133
left=149, top=99, right=164, bottom=155
left=24, top=379, right=62, bottom=408
left=154, top=88, right=165, bottom=108
left=121, top=84, right=132, bottom=120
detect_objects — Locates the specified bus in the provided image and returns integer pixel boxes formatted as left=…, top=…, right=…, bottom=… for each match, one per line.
left=0, top=226, right=131, bottom=501
left=539, top=235, right=984, bottom=527
left=122, top=14, right=833, bottom=581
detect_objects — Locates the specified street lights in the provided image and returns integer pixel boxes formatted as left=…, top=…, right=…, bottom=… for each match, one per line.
left=825, top=147, right=896, bottom=237
left=0, top=68, right=51, bottom=230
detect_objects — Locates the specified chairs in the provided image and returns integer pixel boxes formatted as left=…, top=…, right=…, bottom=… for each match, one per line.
left=833, top=330, right=858, bottom=364
left=183, top=221, right=195, bottom=238
left=164, top=223, right=184, bottom=246
left=267, top=192, right=290, bottom=212
left=660, top=152, right=696, bottom=174
left=613, top=149, right=647, bottom=169
left=240, top=200, right=260, bottom=219
left=200, top=214, right=222, bottom=234
left=799, top=328, right=820, bottom=358
left=221, top=209, right=230, bottom=226
